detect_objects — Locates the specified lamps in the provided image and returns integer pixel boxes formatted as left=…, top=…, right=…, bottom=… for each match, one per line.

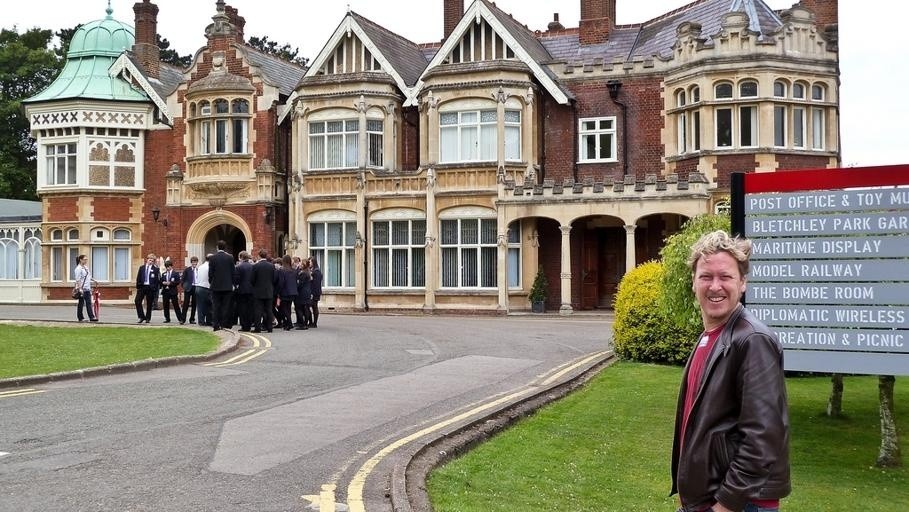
left=264, top=203, right=272, bottom=226
left=150, top=206, right=167, bottom=227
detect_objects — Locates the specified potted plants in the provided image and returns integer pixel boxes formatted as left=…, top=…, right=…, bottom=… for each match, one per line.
left=526, top=262, right=550, bottom=314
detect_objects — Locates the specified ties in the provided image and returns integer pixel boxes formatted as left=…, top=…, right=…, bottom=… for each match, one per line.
left=192, top=269, right=195, bottom=285
left=144, top=266, right=148, bottom=282
left=167, top=270, right=170, bottom=284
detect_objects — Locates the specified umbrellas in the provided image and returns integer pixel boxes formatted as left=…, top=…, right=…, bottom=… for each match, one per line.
left=93, top=282, right=100, bottom=321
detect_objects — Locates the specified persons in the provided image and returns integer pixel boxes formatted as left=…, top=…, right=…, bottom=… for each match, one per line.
left=134, top=240, right=322, bottom=334
left=74, top=255, right=97, bottom=321
left=669, top=230, right=790, bottom=512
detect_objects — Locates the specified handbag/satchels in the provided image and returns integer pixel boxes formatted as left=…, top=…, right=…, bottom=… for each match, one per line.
left=70, top=288, right=80, bottom=299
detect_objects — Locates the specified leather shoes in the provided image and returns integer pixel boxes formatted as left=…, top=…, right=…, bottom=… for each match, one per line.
left=163, top=319, right=317, bottom=333
left=137, top=317, right=145, bottom=323
left=145, top=319, right=149, bottom=323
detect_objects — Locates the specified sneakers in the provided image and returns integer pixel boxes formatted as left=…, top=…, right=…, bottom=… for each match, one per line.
left=89, top=318, right=98, bottom=322
left=79, top=318, right=88, bottom=322
